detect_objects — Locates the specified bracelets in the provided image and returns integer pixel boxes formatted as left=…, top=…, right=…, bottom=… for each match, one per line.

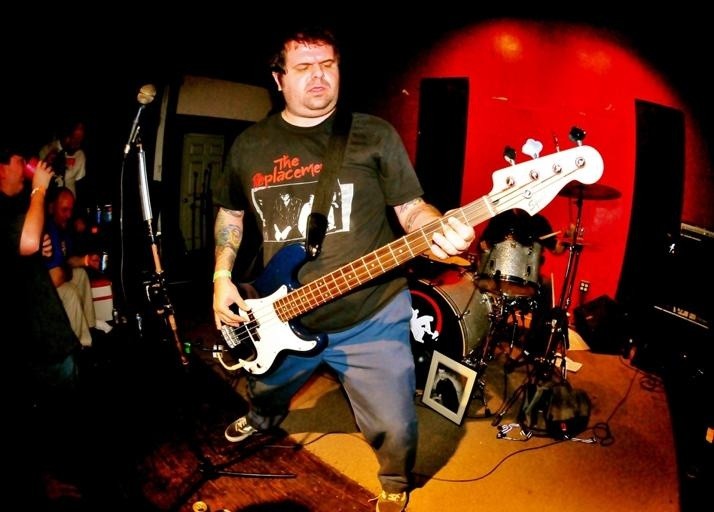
left=212, top=271, right=232, bottom=283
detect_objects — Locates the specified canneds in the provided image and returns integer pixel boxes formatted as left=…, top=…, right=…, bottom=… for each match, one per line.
left=104, top=204, right=112, bottom=223
left=100, top=251, right=108, bottom=271
left=96, top=208, right=101, bottom=224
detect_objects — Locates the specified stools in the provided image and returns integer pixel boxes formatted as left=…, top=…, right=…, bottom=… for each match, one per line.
left=89, top=279, right=115, bottom=323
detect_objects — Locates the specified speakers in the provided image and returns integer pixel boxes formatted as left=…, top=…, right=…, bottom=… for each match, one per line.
left=615, top=98, right=686, bottom=332
left=416, top=78, right=470, bottom=271
left=573, top=295, right=632, bottom=354
left=648, top=222, right=714, bottom=332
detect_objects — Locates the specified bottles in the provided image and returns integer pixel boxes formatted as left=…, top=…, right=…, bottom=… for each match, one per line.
left=113, top=308, right=119, bottom=324
left=86, top=204, right=113, bottom=224
left=191, top=501, right=208, bottom=512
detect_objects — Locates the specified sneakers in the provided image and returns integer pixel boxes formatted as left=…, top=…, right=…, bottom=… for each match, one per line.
left=368, top=488, right=409, bottom=512
left=224, top=416, right=259, bottom=443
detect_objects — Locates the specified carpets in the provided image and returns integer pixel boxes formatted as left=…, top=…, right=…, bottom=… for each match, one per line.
left=182, top=305, right=679, bottom=512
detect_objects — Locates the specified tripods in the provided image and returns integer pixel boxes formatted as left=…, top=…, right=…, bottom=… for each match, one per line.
left=135, top=137, right=297, bottom=512
left=463, top=185, right=585, bottom=439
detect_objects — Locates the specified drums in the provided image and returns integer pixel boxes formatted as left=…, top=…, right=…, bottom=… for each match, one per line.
left=477, top=232, right=546, bottom=297
left=405, top=265, right=493, bottom=395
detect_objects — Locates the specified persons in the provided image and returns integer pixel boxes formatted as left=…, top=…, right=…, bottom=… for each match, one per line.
left=0, top=116, right=101, bottom=393
left=211, top=27, right=477, bottom=512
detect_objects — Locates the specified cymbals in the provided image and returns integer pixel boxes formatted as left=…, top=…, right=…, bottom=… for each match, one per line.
left=420, top=250, right=471, bottom=266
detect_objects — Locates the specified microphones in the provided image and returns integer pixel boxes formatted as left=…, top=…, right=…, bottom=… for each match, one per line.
left=123, top=84, right=156, bottom=158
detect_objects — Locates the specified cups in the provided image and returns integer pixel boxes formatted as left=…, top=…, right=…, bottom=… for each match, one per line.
left=24, top=157, right=37, bottom=181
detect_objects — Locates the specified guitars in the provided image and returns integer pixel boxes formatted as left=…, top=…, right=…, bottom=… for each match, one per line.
left=215, top=146, right=605, bottom=377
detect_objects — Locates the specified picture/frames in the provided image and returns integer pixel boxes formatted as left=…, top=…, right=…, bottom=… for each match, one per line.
left=420, top=349, right=477, bottom=428
